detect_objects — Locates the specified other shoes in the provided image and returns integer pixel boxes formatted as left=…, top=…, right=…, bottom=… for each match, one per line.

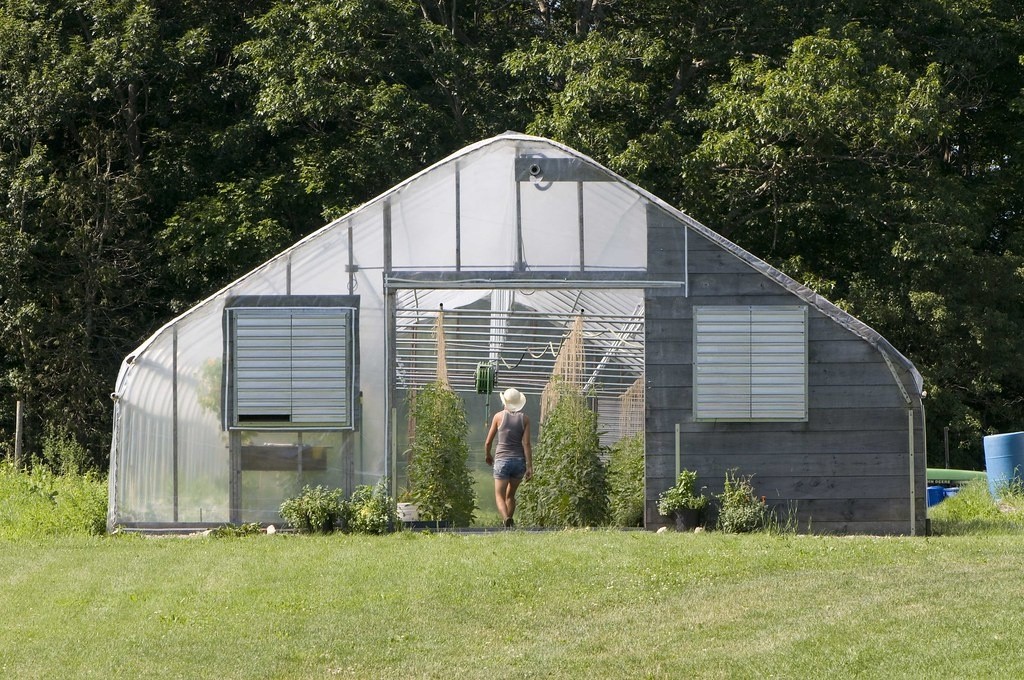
left=503, top=518, right=515, bottom=532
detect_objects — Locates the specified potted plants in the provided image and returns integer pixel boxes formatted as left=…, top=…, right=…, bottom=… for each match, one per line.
left=279, top=483, right=348, bottom=535
left=658, top=469, right=709, bottom=532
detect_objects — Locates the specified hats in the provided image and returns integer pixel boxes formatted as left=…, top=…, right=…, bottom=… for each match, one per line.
left=499, top=388, right=526, bottom=412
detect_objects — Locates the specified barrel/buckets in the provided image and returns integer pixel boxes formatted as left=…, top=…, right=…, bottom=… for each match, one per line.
left=397, top=503, right=418, bottom=521
left=983, top=431, right=1024, bottom=501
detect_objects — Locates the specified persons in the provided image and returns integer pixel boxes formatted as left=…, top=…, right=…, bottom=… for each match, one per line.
left=484, top=387, right=533, bottom=527
